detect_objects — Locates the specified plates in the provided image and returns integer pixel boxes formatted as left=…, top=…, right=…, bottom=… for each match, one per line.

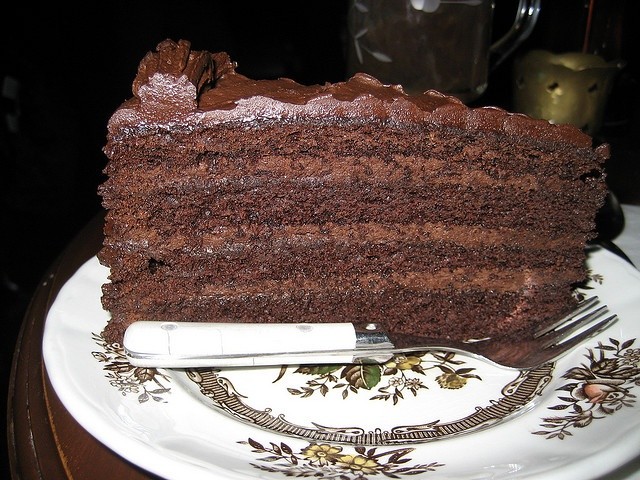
left=42, top=254, right=640, bottom=479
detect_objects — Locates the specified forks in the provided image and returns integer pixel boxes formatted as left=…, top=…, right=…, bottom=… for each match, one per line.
left=124, top=296, right=618, bottom=370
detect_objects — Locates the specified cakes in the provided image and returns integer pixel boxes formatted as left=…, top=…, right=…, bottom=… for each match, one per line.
left=96, top=38, right=611, bottom=346
